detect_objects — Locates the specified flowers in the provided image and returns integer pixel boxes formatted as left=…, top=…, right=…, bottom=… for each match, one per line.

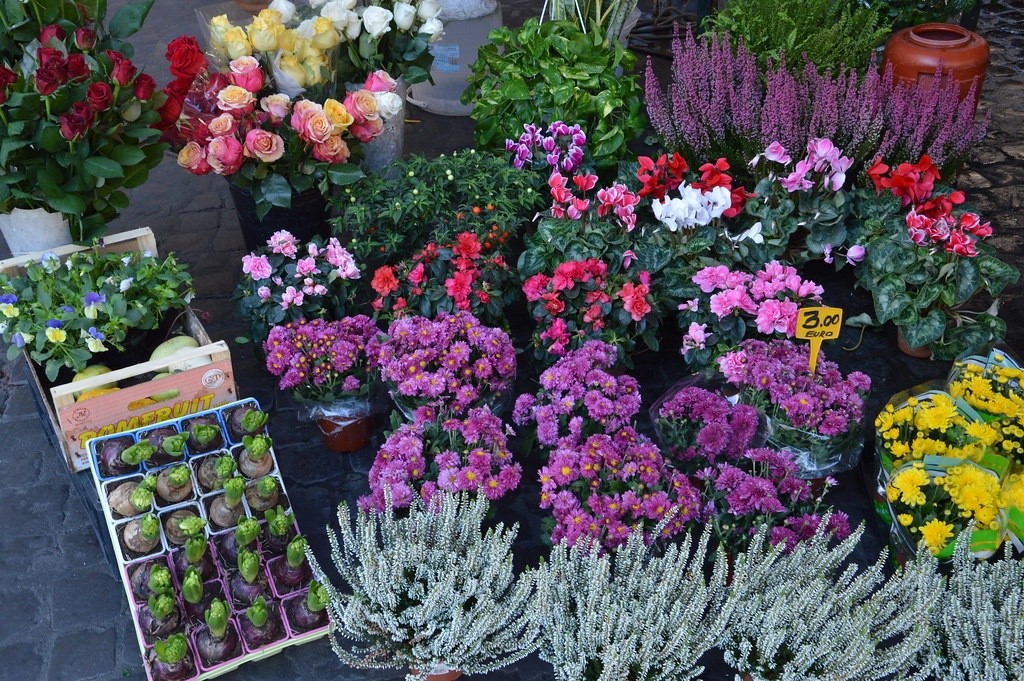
left=0, top=0, right=1024, bottom=570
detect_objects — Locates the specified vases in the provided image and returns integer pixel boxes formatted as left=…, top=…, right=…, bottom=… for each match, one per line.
left=883, top=22, right=991, bottom=126
left=224, top=170, right=334, bottom=257
left=312, top=397, right=371, bottom=452
left=896, top=322, right=931, bottom=360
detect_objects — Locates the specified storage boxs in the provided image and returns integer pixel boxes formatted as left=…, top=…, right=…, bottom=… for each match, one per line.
left=0, top=224, right=238, bottom=477
left=87, top=396, right=340, bottom=681
left=85, top=398, right=335, bottom=681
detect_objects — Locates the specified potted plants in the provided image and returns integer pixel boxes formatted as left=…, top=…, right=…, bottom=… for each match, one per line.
left=146, top=462, right=198, bottom=512
left=199, top=489, right=250, bottom=537
left=127, top=535, right=335, bottom=681
left=100, top=473, right=155, bottom=522
left=211, top=530, right=265, bottom=576
left=111, top=514, right=166, bottom=566
left=90, top=433, right=142, bottom=483
left=219, top=398, right=267, bottom=446
left=241, top=475, right=292, bottom=525
left=157, top=501, right=210, bottom=553
left=176, top=409, right=227, bottom=458
left=135, top=422, right=186, bottom=472
left=255, top=519, right=302, bottom=563
left=305, top=481, right=548, bottom=681
left=228, top=442, right=278, bottom=482
left=188, top=449, right=240, bottom=497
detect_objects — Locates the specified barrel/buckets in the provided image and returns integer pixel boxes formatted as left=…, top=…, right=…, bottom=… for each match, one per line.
left=880, top=22, right=989, bottom=120
left=405, top=1, right=505, bottom=117
